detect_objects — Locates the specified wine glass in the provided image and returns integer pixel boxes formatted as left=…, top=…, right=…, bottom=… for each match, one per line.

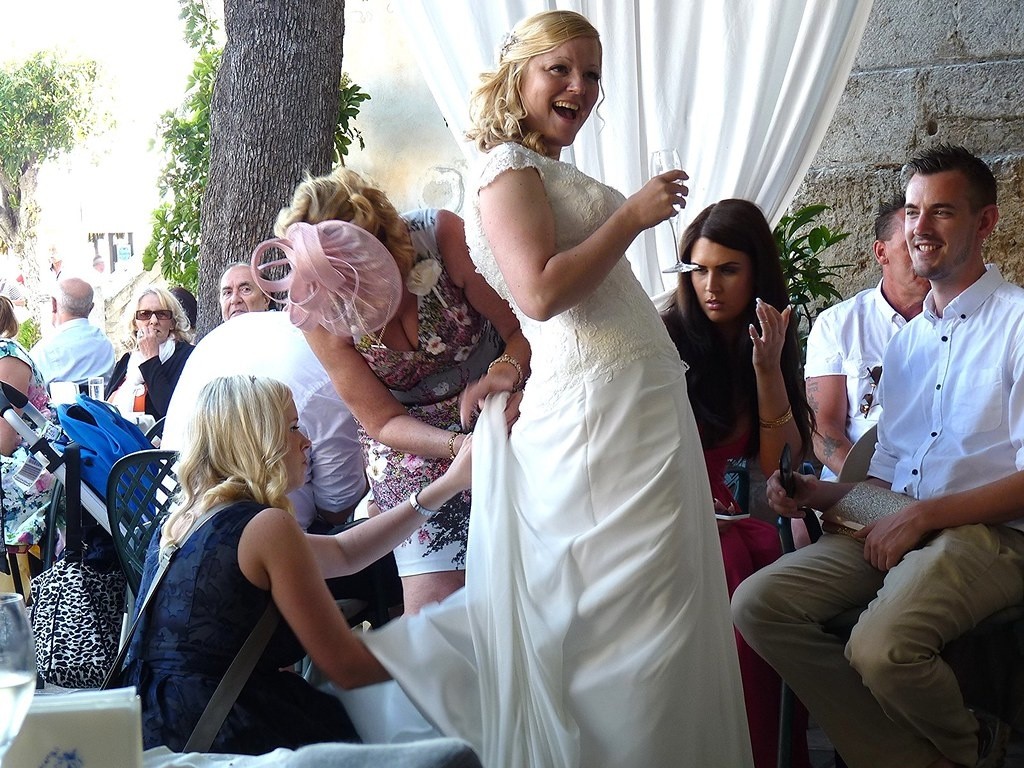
left=650, top=148, right=699, bottom=274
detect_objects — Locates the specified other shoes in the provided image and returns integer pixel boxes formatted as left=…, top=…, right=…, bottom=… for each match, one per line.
left=975, top=711, right=1011, bottom=767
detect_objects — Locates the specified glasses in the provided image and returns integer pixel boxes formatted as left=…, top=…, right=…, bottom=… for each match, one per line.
left=135, top=310, right=174, bottom=322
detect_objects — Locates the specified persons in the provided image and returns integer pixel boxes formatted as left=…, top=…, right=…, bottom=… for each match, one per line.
left=730, top=142, right=1024, bottom=768
left=658, top=198, right=812, bottom=768
left=0, top=262, right=279, bottom=607
left=304, top=10, right=759, bottom=768
left=115, top=167, right=532, bottom=756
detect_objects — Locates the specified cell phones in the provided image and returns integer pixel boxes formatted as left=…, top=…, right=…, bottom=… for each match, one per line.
left=715, top=510, right=750, bottom=521
left=780, top=442, right=796, bottom=499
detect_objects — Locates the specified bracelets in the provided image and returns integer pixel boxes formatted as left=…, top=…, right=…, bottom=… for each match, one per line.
left=487, top=353, right=523, bottom=389
left=760, top=404, right=793, bottom=429
left=449, top=433, right=467, bottom=461
left=409, top=487, right=437, bottom=517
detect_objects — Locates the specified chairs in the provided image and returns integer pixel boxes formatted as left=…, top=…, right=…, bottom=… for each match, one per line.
left=777, top=508, right=1024, bottom=767
left=832, top=424, right=878, bottom=485
left=106, top=447, right=184, bottom=600
left=2, top=443, right=83, bottom=603
left=144, top=416, right=166, bottom=449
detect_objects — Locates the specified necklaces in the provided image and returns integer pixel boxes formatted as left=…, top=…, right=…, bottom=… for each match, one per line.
left=352, top=292, right=394, bottom=349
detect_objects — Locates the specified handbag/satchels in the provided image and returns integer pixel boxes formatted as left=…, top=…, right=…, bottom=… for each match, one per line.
left=30, top=543, right=129, bottom=688
left=819, top=481, right=921, bottom=543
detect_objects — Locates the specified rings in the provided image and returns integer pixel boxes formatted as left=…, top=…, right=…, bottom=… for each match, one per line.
left=759, top=320, right=769, bottom=324
left=137, top=331, right=144, bottom=338
left=750, top=333, right=759, bottom=340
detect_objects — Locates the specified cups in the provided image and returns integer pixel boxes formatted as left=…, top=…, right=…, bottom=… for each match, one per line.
left=89, top=376, right=105, bottom=402
left=1, top=592, right=36, bottom=766
